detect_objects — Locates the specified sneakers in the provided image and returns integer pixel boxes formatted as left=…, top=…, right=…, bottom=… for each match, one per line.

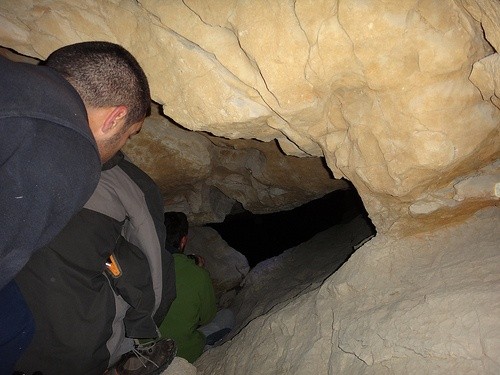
left=116, top=339, right=175, bottom=375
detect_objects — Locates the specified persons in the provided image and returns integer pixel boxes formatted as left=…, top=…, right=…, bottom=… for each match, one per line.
left=139, top=212, right=215, bottom=364
left=1, top=41, right=151, bottom=375
left=15, top=149, right=178, bottom=375
